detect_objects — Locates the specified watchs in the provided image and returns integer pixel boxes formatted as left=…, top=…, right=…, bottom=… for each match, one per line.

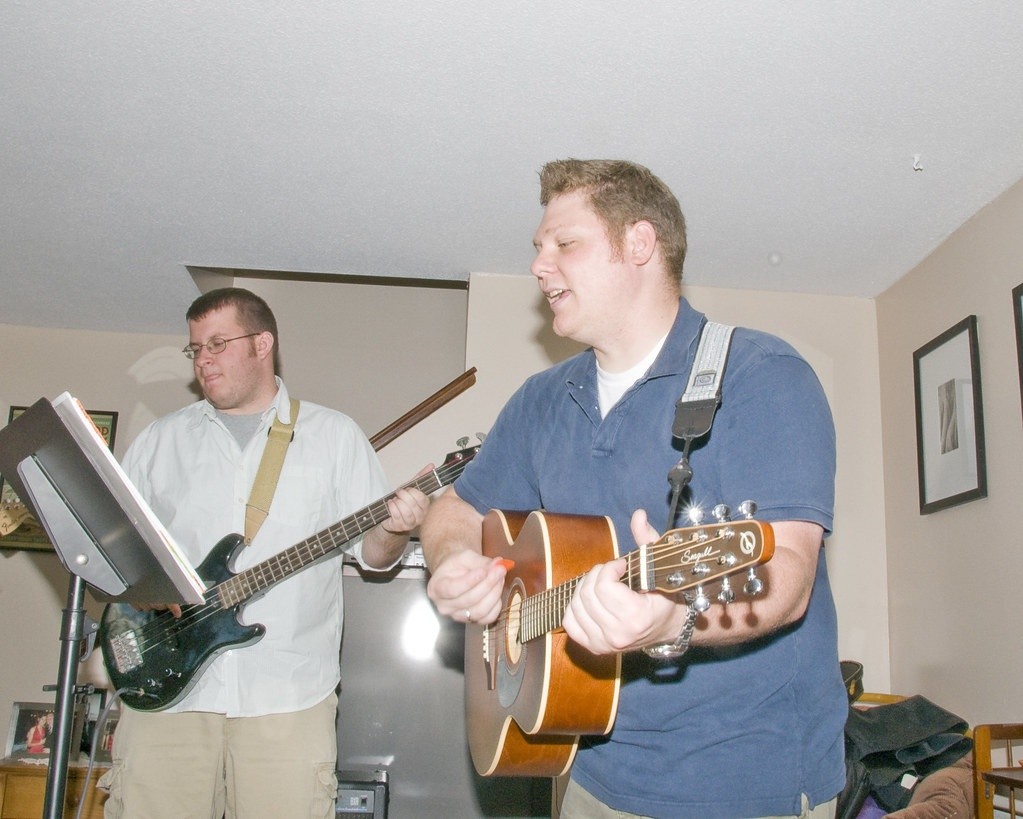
left=643, top=605, right=699, bottom=660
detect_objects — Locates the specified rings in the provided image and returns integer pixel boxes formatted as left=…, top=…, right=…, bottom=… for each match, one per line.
left=466, top=610, right=473, bottom=624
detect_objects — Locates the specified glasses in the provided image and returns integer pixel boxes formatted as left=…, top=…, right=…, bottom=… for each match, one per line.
left=182, top=332, right=261, bottom=360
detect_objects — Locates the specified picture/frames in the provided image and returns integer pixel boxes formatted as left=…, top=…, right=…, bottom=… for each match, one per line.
left=73, top=687, right=121, bottom=763
left=6, top=702, right=56, bottom=764
left=0, top=403, right=117, bottom=553
left=910, top=314, right=985, bottom=515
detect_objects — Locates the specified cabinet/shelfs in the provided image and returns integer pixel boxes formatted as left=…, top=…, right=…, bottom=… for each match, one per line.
left=0, top=761, right=112, bottom=819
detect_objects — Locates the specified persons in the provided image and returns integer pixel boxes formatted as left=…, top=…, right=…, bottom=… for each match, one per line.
left=96, top=289, right=434, bottom=819
left=420, top=158, right=849, bottom=819
left=27, top=714, right=53, bottom=753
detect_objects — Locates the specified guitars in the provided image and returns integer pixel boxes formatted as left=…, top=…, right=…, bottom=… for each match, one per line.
left=459, top=505, right=774, bottom=780
left=89, top=434, right=498, bottom=712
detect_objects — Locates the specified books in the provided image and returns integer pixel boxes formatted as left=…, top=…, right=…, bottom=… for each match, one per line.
left=49, top=391, right=207, bottom=605
